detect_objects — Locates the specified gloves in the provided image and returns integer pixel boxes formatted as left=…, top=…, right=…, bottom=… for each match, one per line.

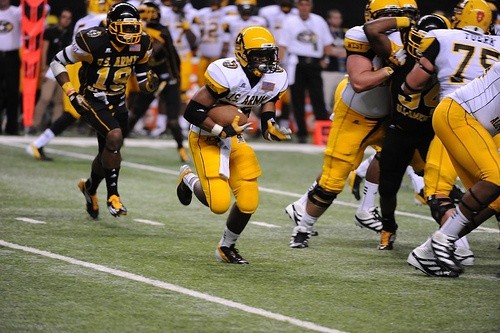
left=68, top=93, right=91, bottom=116
left=221, top=116, right=253, bottom=139
left=268, top=118, right=293, bottom=141
left=385, top=49, right=408, bottom=71
left=146, top=70, right=161, bottom=92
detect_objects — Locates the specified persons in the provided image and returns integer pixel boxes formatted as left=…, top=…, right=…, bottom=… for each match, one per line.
left=286, top=0, right=500, bottom=278
left=50, top=3, right=158, bottom=218
left=177, top=26, right=291, bottom=264
left=326, top=11, right=350, bottom=72
left=278, top=0, right=334, bottom=140
left=0, top=0, right=300, bottom=163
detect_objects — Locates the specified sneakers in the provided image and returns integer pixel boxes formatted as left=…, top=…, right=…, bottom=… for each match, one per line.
left=27, top=144, right=53, bottom=161
left=177, top=148, right=188, bottom=164
left=107, top=195, right=127, bottom=216
left=216, top=242, right=249, bottom=265
left=408, top=247, right=459, bottom=278
left=431, top=232, right=464, bottom=274
left=290, top=225, right=311, bottom=249
left=454, top=253, right=475, bottom=266
left=78, top=179, right=99, bottom=219
left=355, top=207, right=398, bottom=232
left=377, top=229, right=396, bottom=250
left=287, top=203, right=318, bottom=236
left=349, top=171, right=362, bottom=200
left=177, top=165, right=193, bottom=206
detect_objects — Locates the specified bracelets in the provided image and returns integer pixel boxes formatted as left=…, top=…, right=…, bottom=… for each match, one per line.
left=67, top=88, right=74, bottom=96
left=62, top=82, right=74, bottom=92
left=211, top=124, right=223, bottom=135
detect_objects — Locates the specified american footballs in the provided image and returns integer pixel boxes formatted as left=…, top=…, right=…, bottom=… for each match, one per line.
left=208, top=105, right=248, bottom=129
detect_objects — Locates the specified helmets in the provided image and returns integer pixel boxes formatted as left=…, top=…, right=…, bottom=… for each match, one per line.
left=237, top=0, right=257, bottom=15
left=453, top=0, right=492, bottom=34
left=236, top=26, right=284, bottom=76
left=365, top=0, right=419, bottom=30
left=107, top=3, right=142, bottom=44
left=406, top=13, right=452, bottom=58
